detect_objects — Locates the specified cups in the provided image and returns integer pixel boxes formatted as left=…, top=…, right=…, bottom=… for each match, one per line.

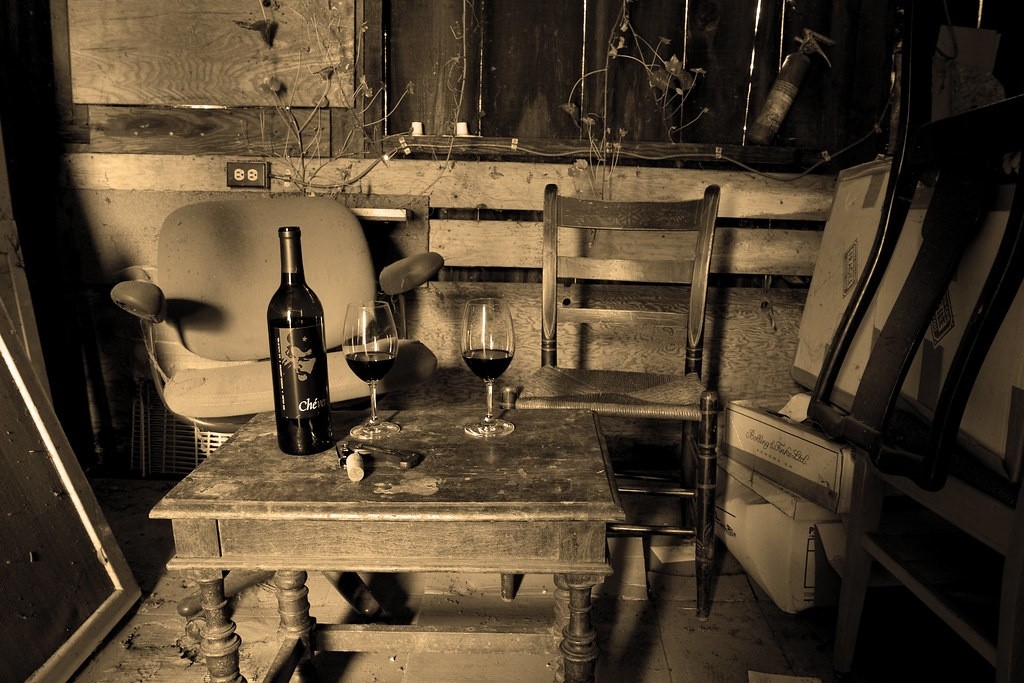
left=457, top=122, right=468, bottom=135
left=412, top=121, right=422, bottom=136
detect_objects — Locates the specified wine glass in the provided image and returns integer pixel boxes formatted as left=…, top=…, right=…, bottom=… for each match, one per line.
left=460, top=297, right=515, bottom=439
left=341, top=300, right=401, bottom=441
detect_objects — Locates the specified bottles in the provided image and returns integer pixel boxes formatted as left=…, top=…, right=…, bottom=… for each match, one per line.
left=266, top=226, right=334, bottom=457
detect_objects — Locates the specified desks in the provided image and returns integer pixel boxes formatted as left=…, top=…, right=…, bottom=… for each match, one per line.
left=147, top=408, right=630, bottom=683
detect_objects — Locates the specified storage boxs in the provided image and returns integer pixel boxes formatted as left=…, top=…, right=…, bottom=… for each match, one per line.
left=711, top=394, right=860, bottom=616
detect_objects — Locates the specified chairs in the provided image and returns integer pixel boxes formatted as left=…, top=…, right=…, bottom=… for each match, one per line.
left=106, top=191, right=439, bottom=622
left=502, top=180, right=721, bottom=623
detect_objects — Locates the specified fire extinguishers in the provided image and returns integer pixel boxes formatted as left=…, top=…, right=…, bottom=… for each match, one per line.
left=746, top=28, right=844, bottom=148
